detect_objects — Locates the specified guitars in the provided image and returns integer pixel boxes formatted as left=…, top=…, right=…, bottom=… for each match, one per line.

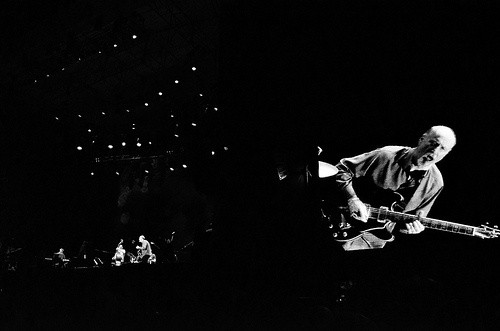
left=319, top=190, right=500, bottom=242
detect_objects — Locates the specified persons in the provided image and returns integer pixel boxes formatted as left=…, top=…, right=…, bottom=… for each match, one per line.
left=57, top=248, right=65, bottom=260
left=333, top=126, right=457, bottom=307
left=112, top=239, right=126, bottom=262
left=136, top=235, right=152, bottom=263
